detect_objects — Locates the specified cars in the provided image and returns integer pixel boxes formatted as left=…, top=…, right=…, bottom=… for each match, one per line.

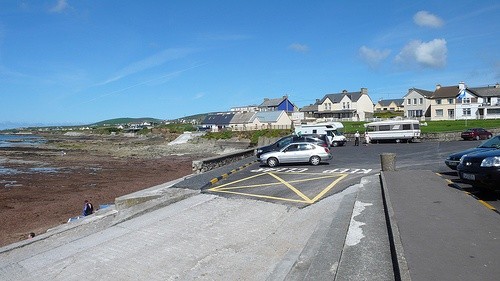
left=257, top=133, right=327, bottom=160
left=444, top=135, right=500, bottom=170
left=261, top=141, right=332, bottom=167
left=456, top=149, right=500, bottom=189
left=461, top=128, right=494, bottom=140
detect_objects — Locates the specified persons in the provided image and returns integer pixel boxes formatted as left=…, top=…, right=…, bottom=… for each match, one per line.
left=83, top=200, right=93, bottom=216
left=354, top=131, right=360, bottom=146
left=28, top=232, right=35, bottom=238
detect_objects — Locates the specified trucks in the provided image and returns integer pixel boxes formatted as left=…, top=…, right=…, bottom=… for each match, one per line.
left=300, top=127, right=347, bottom=147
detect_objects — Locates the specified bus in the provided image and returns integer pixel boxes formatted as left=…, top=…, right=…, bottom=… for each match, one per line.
left=365, top=119, right=420, bottom=142
